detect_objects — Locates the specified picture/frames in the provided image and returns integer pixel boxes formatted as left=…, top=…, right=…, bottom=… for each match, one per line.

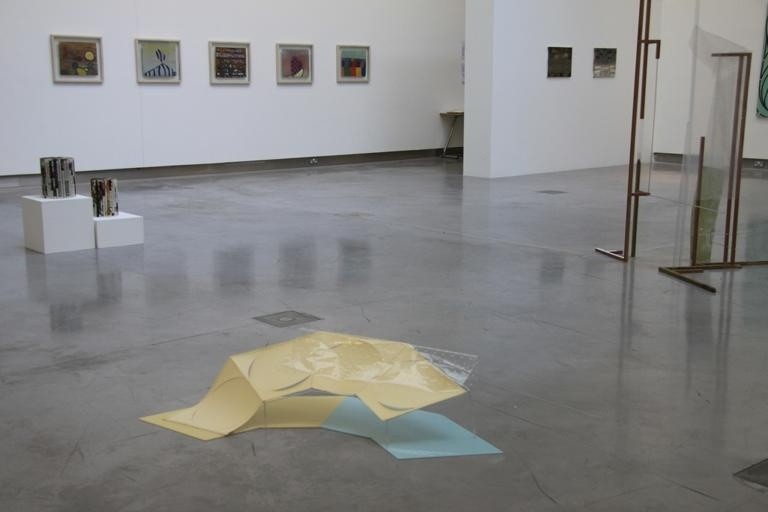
left=135, top=38, right=182, bottom=84
left=336, top=44, right=370, bottom=84
left=208, top=40, right=251, bottom=85
left=49, top=34, right=102, bottom=84
left=275, top=43, right=312, bottom=84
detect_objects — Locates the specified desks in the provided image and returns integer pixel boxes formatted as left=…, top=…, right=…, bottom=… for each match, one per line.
left=441, top=112, right=464, bottom=157
